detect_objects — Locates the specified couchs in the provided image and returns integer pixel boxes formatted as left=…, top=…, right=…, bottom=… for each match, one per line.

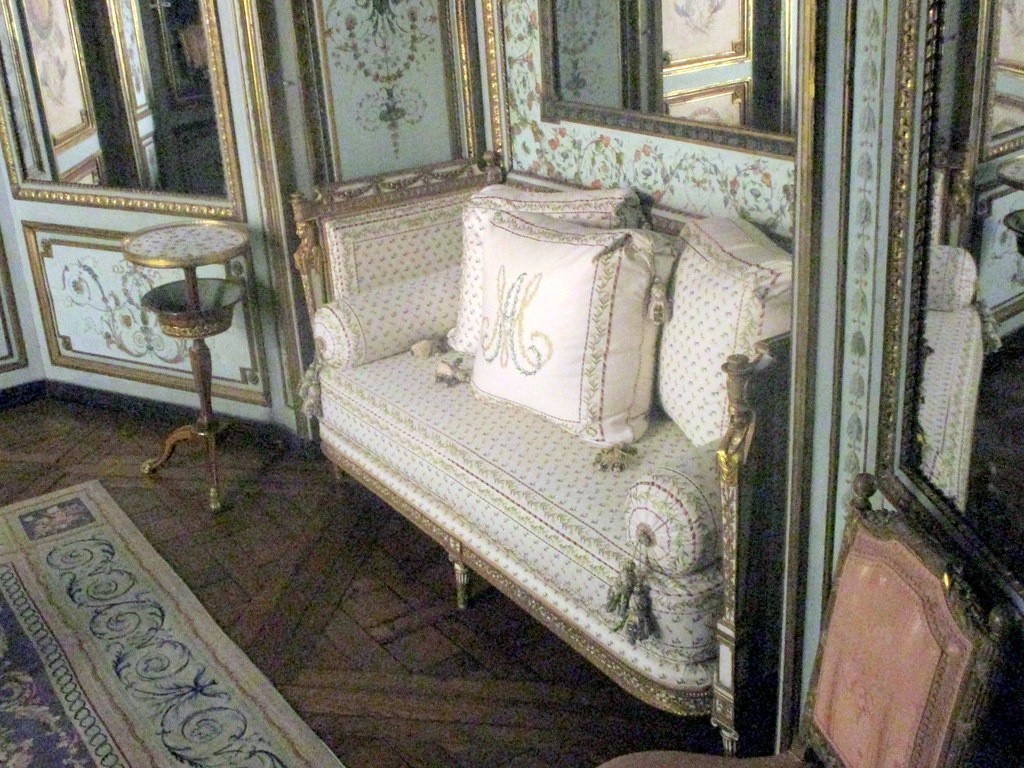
left=291, top=101, right=797, bottom=760
left=918, top=239, right=1001, bottom=519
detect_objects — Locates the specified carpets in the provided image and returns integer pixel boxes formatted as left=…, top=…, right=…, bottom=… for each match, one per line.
left=0, top=479, right=346, bottom=768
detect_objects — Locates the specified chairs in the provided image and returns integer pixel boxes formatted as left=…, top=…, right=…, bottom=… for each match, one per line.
left=591, top=472, right=1024, bottom=767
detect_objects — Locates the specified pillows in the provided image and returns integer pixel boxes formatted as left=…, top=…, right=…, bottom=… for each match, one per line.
left=655, top=218, right=794, bottom=446
left=443, top=184, right=652, bottom=359
left=470, top=209, right=682, bottom=473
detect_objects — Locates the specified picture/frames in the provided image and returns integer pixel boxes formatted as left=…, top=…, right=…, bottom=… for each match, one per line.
left=661, top=78, right=755, bottom=128
left=662, top=0, right=755, bottom=77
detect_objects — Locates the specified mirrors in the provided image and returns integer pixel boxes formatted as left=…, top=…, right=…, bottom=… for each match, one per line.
left=977, top=1, right=1023, bottom=166
left=540, top=1, right=797, bottom=165
left=0, top=0, right=247, bottom=220
left=875, top=0, right=1024, bottom=660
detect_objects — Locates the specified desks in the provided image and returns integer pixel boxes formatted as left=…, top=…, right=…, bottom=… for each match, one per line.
left=121, top=221, right=287, bottom=515
